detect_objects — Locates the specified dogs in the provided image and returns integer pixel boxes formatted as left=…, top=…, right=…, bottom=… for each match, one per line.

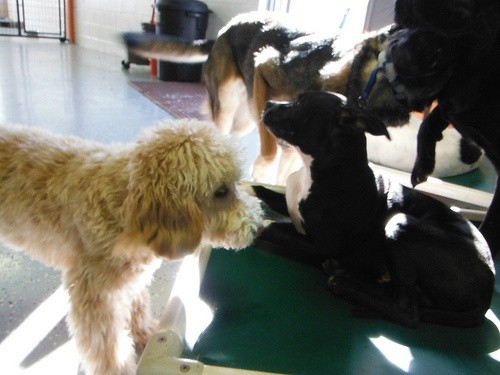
left=0, top=116, right=267, bottom=375
left=249, top=89, right=497, bottom=331
left=387, top=0, right=500, bottom=260
left=200, top=11, right=406, bottom=164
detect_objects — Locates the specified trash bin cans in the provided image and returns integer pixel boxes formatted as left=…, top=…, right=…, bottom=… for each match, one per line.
left=153, top=1, right=215, bottom=84
